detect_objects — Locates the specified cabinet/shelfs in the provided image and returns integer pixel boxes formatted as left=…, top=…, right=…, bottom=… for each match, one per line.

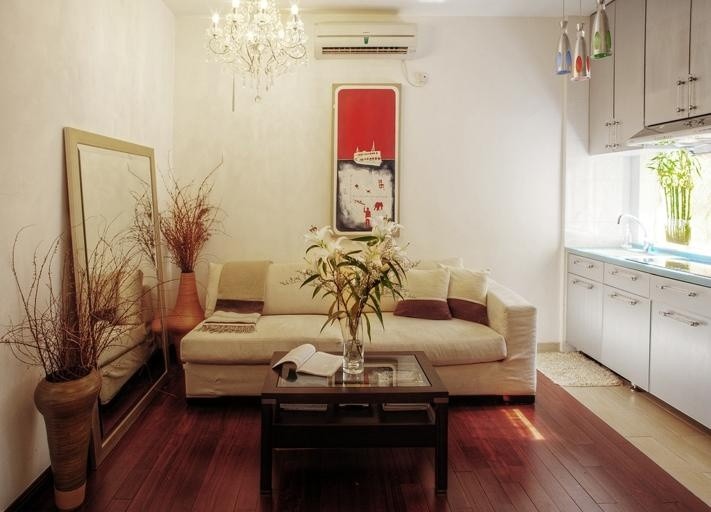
left=646, top=2, right=711, bottom=138
left=562, top=250, right=603, bottom=361
left=647, top=268, right=711, bottom=431
left=586, top=3, right=646, bottom=155
left=606, top=258, right=653, bottom=394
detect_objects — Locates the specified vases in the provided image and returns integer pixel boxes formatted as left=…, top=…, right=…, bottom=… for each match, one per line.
left=151, top=313, right=163, bottom=344
left=337, top=309, right=366, bottom=376
left=30, top=366, right=109, bottom=512
left=171, top=272, right=207, bottom=368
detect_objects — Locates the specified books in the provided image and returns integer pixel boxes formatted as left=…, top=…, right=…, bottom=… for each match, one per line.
left=271, top=342, right=347, bottom=378
left=377, top=367, right=431, bottom=410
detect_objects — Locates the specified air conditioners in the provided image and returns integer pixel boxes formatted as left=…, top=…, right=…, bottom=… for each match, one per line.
left=313, top=25, right=418, bottom=57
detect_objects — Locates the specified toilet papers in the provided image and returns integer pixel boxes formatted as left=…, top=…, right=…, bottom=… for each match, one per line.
left=619, top=223, right=633, bottom=248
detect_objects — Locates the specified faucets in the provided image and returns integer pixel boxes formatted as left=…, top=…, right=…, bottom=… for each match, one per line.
left=616, top=213, right=649, bottom=251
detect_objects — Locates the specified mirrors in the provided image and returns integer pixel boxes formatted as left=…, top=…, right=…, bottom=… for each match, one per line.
left=63, top=123, right=167, bottom=459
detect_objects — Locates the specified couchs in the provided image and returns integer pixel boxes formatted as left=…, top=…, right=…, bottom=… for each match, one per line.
left=92, top=263, right=151, bottom=406
left=178, top=259, right=541, bottom=412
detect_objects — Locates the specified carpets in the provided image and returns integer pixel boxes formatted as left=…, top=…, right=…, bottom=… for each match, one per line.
left=536, top=346, right=625, bottom=387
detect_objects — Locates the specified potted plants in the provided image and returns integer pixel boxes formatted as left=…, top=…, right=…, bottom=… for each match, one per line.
left=650, top=146, right=704, bottom=246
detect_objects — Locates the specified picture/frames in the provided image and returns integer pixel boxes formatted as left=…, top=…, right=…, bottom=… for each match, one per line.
left=332, top=83, right=403, bottom=238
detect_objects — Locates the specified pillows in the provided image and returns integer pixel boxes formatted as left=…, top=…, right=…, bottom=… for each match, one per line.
left=393, top=266, right=452, bottom=319
left=447, top=264, right=494, bottom=325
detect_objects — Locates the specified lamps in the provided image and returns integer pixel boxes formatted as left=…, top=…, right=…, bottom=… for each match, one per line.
left=553, top=0, right=612, bottom=85
left=202, top=3, right=307, bottom=108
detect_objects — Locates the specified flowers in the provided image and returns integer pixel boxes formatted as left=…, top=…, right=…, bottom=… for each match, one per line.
left=287, top=223, right=420, bottom=363
